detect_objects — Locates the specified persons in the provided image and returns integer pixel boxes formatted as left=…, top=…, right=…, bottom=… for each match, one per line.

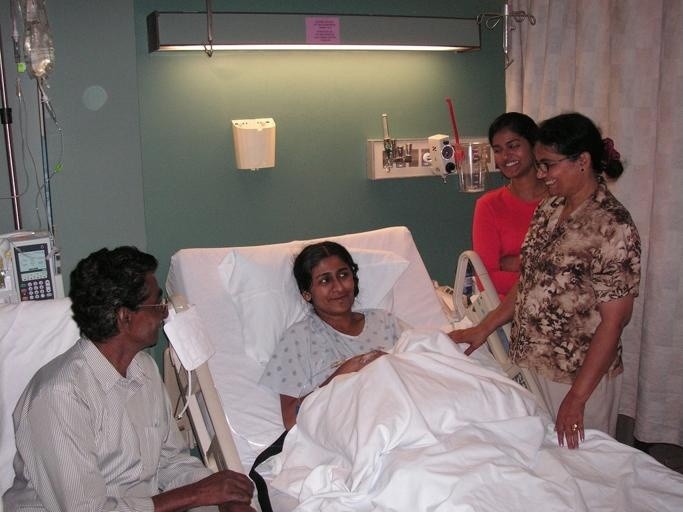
left=443, top=112, right=643, bottom=451
left=471, top=112, right=551, bottom=359
left=260, top=241, right=415, bottom=435
left=0, top=246, right=257, bottom=512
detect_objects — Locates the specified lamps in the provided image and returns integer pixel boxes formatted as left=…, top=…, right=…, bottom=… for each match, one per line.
left=147, top=12, right=481, bottom=53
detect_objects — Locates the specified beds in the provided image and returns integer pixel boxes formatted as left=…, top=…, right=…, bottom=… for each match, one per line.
left=162, top=227, right=682, bottom=512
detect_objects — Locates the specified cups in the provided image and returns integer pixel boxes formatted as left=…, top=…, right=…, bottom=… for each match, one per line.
left=452, top=140, right=489, bottom=194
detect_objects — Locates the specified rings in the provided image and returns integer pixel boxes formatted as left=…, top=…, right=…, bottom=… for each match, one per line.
left=572, top=424, right=579, bottom=432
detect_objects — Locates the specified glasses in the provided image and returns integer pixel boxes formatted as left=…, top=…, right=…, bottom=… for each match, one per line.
left=136, top=298, right=169, bottom=318
left=534, top=156, right=569, bottom=172
